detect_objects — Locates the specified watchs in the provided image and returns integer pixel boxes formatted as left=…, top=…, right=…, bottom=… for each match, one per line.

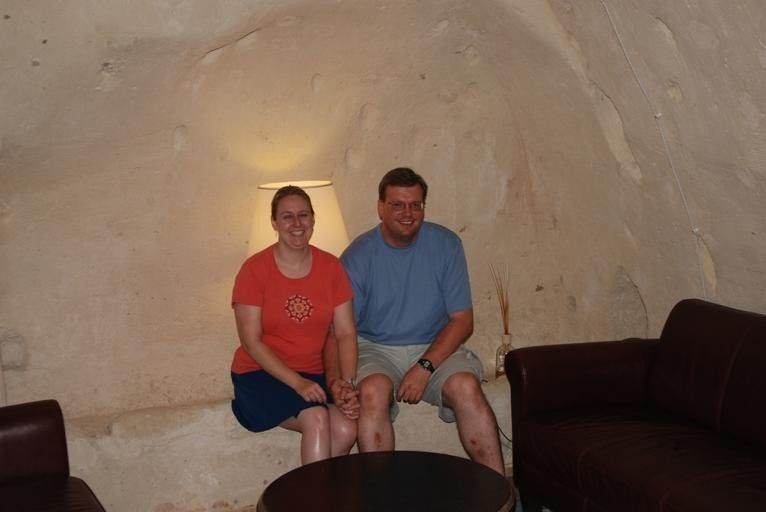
left=417, top=358, right=435, bottom=374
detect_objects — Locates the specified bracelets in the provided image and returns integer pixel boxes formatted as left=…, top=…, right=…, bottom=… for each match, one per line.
left=329, top=378, right=336, bottom=392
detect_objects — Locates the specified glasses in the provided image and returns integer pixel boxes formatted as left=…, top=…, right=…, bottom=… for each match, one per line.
left=382, top=200, right=425, bottom=212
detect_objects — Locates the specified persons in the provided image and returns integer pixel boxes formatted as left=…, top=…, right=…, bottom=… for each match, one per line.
left=230, top=184, right=358, bottom=465
left=328, top=167, right=507, bottom=478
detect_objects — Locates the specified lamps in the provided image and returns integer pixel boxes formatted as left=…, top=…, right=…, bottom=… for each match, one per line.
left=245, top=181, right=352, bottom=257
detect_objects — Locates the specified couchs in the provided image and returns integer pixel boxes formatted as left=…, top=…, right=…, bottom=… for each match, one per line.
left=503, top=296, right=765, bottom=511
left=0, top=398, right=107, bottom=512
